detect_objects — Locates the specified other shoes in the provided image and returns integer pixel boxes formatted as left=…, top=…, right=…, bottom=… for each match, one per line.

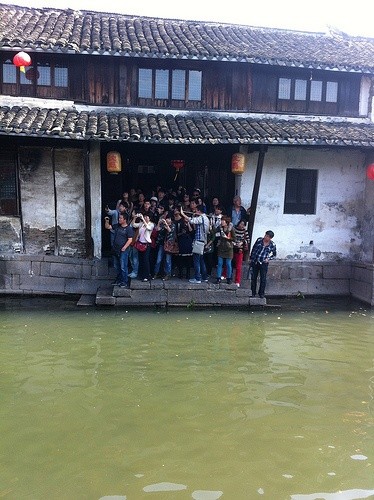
left=162, top=274, right=172, bottom=281
left=215, top=278, right=221, bottom=284
left=111, top=279, right=121, bottom=285
left=236, top=282, right=241, bottom=287
left=142, top=274, right=151, bottom=281
left=199, top=277, right=209, bottom=283
left=120, top=283, right=128, bottom=287
left=221, top=275, right=226, bottom=280
left=259, top=294, right=264, bottom=298
left=151, top=272, right=158, bottom=280
left=128, top=271, right=138, bottom=278
left=189, top=278, right=201, bottom=283
left=227, top=278, right=232, bottom=284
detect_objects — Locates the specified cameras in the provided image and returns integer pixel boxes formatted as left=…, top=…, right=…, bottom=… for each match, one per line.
left=137, top=213, right=141, bottom=217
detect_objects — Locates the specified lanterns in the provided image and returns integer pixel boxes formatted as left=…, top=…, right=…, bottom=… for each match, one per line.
left=11, top=51, right=32, bottom=72
left=365, top=161, right=374, bottom=182
left=105, top=149, right=124, bottom=176
left=229, top=149, right=247, bottom=177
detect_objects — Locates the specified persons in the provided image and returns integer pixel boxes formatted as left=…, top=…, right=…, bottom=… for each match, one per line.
left=250, top=231, right=276, bottom=298
left=98, top=179, right=250, bottom=287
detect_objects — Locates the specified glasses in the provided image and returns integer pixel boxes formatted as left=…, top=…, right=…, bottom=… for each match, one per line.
left=193, top=193, right=199, bottom=196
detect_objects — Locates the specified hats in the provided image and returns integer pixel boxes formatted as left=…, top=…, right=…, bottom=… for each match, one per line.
left=151, top=197, right=158, bottom=203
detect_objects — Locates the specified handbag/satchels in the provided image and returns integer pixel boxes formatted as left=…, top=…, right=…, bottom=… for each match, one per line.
left=134, top=240, right=148, bottom=251
left=243, top=231, right=249, bottom=253
left=110, top=243, right=122, bottom=256
left=164, top=240, right=179, bottom=253
left=192, top=240, right=204, bottom=255
